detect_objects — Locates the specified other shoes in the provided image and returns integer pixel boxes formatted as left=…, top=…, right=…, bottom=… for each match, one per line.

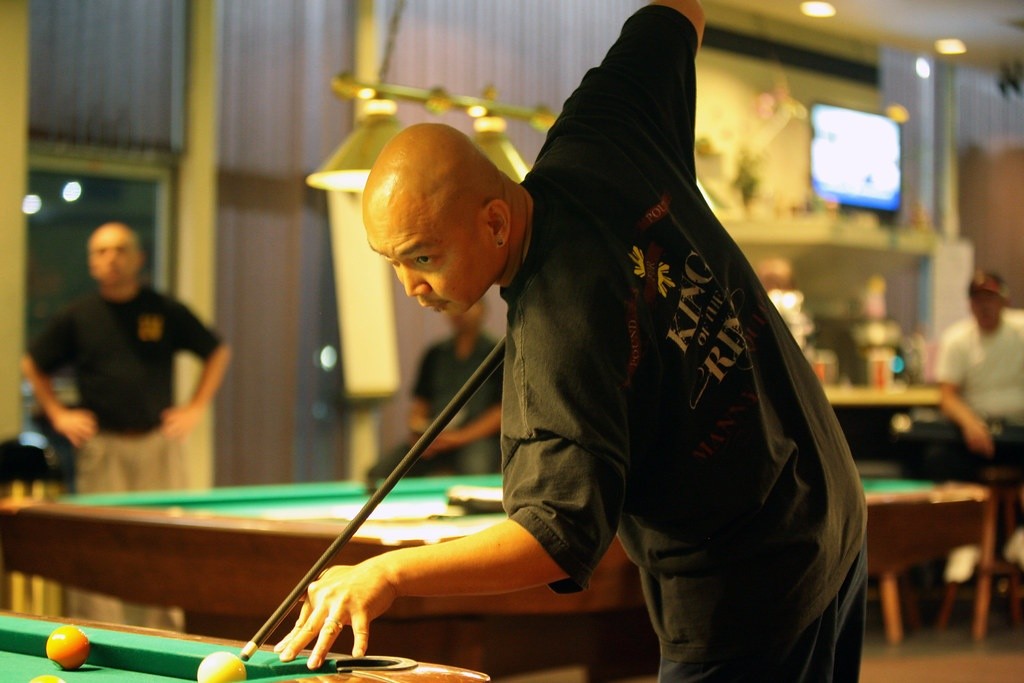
left=943, top=544, right=982, bottom=585
left=1003, top=526, right=1024, bottom=569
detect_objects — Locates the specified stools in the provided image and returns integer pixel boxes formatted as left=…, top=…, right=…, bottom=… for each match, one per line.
left=934, top=465, right=1024, bottom=646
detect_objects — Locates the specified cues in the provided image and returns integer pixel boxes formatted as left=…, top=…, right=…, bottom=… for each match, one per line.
left=237, top=333, right=509, bottom=663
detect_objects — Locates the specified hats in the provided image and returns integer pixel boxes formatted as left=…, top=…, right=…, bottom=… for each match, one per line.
left=969, top=273, right=1011, bottom=300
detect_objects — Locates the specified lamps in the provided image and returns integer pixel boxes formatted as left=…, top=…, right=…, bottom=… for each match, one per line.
left=302, top=96, right=405, bottom=193
left=470, top=117, right=532, bottom=184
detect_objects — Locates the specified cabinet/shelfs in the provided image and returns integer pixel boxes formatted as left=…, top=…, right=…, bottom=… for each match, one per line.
left=712, top=212, right=952, bottom=410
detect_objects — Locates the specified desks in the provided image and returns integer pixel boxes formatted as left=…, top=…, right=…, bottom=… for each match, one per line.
left=0, top=468, right=989, bottom=683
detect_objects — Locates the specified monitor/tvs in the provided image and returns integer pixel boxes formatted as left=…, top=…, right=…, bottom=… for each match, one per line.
left=808, top=101, right=902, bottom=211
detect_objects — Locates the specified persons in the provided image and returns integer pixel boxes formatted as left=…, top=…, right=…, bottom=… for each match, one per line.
left=19, top=221, right=231, bottom=634
left=273, top=0, right=868, bottom=683
left=898, top=270, right=1024, bottom=583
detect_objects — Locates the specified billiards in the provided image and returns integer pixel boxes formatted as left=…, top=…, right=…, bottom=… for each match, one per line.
left=196, top=650, right=248, bottom=683
left=43, top=623, right=91, bottom=670
left=27, top=675, right=67, bottom=683
